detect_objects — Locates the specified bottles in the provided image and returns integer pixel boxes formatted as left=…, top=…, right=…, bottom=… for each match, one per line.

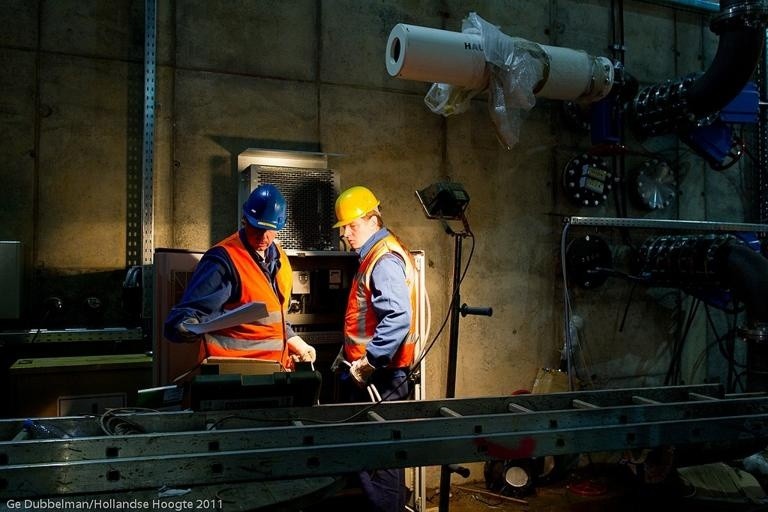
left=24, top=417, right=61, bottom=441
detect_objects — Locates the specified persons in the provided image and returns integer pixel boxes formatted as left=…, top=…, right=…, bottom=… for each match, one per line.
left=331, top=186, right=418, bottom=512
left=163, top=184, right=316, bottom=372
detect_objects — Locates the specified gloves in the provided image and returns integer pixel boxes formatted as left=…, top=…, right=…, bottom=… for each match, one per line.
left=348, top=354, right=380, bottom=390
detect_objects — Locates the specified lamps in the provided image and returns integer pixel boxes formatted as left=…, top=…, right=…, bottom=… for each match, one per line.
left=414, top=181, right=471, bottom=220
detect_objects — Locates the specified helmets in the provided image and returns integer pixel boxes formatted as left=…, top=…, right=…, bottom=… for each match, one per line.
left=242, top=184, right=287, bottom=231
left=331, top=186, right=381, bottom=229
